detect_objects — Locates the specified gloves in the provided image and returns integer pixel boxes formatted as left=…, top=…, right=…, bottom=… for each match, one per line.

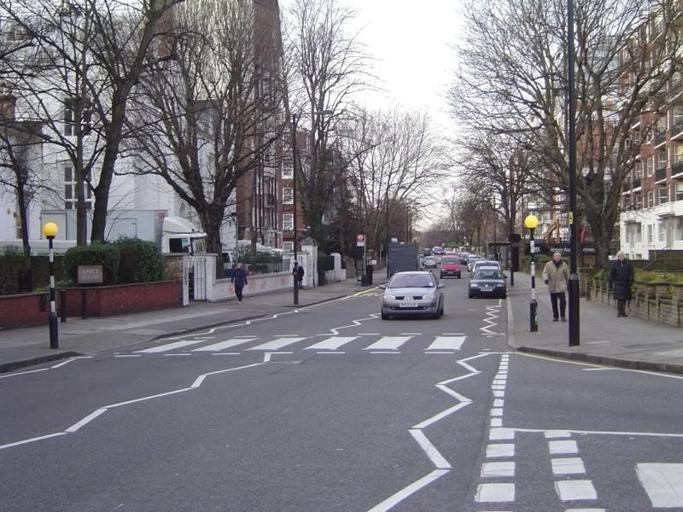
left=544, top=280, right=548, bottom=284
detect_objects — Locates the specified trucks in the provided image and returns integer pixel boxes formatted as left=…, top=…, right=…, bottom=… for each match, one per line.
left=40, top=209, right=208, bottom=279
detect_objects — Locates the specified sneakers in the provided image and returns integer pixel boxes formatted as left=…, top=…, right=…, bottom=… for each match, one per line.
left=617, top=312, right=627, bottom=317
left=553, top=317, right=567, bottom=321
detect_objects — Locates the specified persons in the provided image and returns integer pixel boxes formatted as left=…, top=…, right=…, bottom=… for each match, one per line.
left=607, top=250, right=634, bottom=317
left=543, top=252, right=569, bottom=321
left=293, top=262, right=304, bottom=288
left=230, top=263, right=249, bottom=302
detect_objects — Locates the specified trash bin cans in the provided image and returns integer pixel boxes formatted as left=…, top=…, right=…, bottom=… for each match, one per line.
left=362, top=275, right=369, bottom=286
left=319, top=272, right=324, bottom=286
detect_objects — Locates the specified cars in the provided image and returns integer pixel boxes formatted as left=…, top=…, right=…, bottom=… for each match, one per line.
left=379, top=241, right=507, bottom=319
left=222, top=251, right=249, bottom=275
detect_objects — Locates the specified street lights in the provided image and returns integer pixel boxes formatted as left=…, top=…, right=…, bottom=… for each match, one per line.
left=525, top=215, right=538, bottom=331
left=292, top=110, right=334, bottom=302
left=42, top=223, right=58, bottom=350
